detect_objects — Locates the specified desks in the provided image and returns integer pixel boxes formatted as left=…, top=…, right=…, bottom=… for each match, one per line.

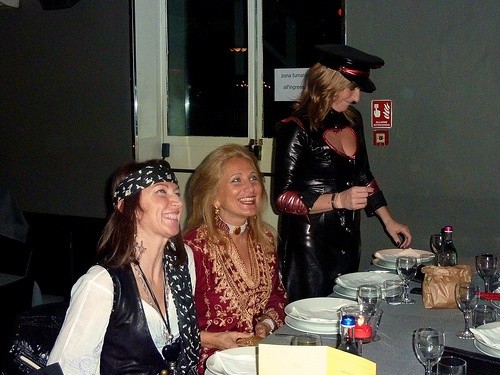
left=257, top=258, right=500, bottom=375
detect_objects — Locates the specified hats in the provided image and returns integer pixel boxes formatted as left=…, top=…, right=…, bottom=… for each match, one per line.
left=313, top=44, right=385, bottom=93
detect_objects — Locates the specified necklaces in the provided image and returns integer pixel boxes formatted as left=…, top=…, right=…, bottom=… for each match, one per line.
left=133, top=255, right=180, bottom=375
left=220, top=218, right=248, bottom=235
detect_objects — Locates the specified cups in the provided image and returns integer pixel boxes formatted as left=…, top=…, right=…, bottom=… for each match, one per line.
left=420, top=253, right=450, bottom=266
left=437, top=356, right=467, bottom=375
left=290, top=333, right=322, bottom=346
left=473, top=304, right=496, bottom=328
left=430, top=233, right=446, bottom=253
left=383, top=279, right=404, bottom=306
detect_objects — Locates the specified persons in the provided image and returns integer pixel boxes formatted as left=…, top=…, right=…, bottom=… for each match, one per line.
left=271, top=47, right=412, bottom=304
left=47, top=159, right=200, bottom=375
left=180, top=144, right=290, bottom=375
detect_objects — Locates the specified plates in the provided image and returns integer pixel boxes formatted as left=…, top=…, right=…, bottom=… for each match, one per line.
left=206, top=346, right=258, bottom=375
left=490, top=287, right=500, bottom=310
left=285, top=316, right=340, bottom=335
left=333, top=271, right=402, bottom=299
left=284, top=298, right=358, bottom=325
left=474, top=321, right=500, bottom=358
left=372, top=249, right=435, bottom=270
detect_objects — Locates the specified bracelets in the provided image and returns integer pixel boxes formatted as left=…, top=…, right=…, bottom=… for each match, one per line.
left=263, top=318, right=276, bottom=334
left=332, top=192, right=337, bottom=210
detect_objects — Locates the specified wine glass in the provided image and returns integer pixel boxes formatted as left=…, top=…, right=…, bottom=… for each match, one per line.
left=412, top=328, right=445, bottom=375
left=396, top=255, right=417, bottom=304
left=455, top=282, right=480, bottom=340
left=340, top=304, right=384, bottom=357
left=357, top=283, right=382, bottom=342
left=475, top=253, right=499, bottom=293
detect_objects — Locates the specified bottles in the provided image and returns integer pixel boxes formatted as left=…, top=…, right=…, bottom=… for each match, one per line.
left=437, top=226, right=458, bottom=267
left=337, top=314, right=361, bottom=356
